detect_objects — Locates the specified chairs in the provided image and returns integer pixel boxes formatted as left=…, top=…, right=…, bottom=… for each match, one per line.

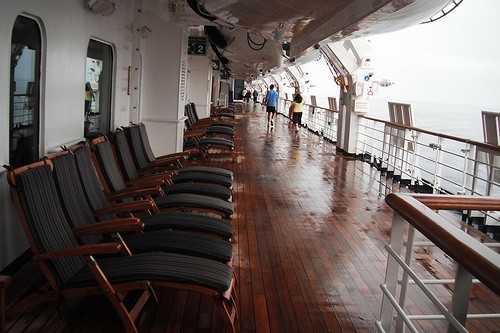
left=4, top=102, right=238, bottom=333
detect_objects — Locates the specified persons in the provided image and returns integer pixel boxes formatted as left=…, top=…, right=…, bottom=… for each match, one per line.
left=84, top=82, right=95, bottom=122
left=265, top=84, right=278, bottom=127
left=246, top=90, right=251, bottom=103
left=253, top=91, right=258, bottom=103
left=290, top=94, right=303, bottom=132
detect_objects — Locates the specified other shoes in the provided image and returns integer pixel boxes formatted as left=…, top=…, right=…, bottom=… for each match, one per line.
left=295, top=129, right=299, bottom=132
left=270, top=120, right=273, bottom=126
